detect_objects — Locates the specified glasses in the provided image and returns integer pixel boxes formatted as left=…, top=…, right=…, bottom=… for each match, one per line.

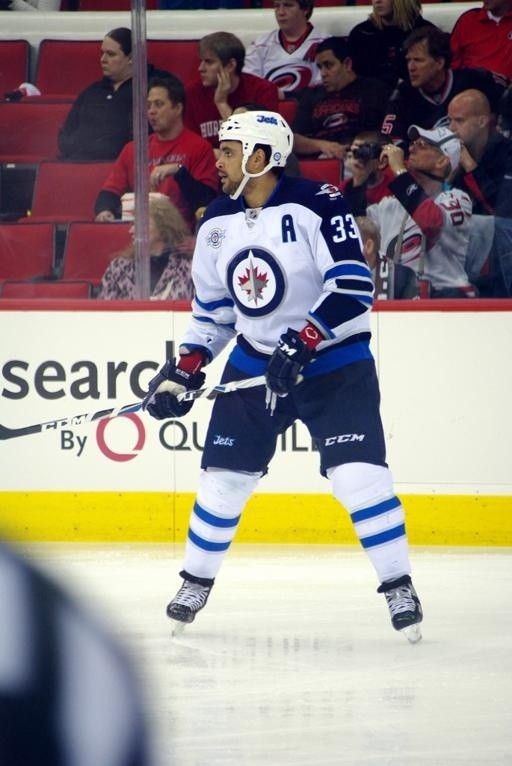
left=412, top=140, right=443, bottom=156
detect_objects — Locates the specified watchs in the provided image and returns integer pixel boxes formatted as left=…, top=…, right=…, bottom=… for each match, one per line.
left=178, top=164, right=186, bottom=170
left=395, top=168, right=408, bottom=177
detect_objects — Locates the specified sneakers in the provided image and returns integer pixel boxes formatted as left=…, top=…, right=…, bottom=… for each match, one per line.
left=377, top=575, right=423, bottom=631
left=167, top=571, right=215, bottom=622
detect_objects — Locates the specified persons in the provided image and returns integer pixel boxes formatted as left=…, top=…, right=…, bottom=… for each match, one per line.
left=450, top=0, right=512, bottom=79
left=183, top=31, right=280, bottom=159
left=292, top=37, right=384, bottom=164
left=381, top=28, right=511, bottom=142
left=341, top=125, right=475, bottom=299
left=447, top=89, right=512, bottom=216
left=59, top=28, right=156, bottom=163
left=354, top=217, right=419, bottom=299
left=350, top=1, right=448, bottom=72
left=338, top=131, right=394, bottom=205
left=145, top=110, right=442, bottom=642
left=243, top=0, right=331, bottom=99
left=95, top=78, right=217, bottom=222
left=96, top=194, right=196, bottom=302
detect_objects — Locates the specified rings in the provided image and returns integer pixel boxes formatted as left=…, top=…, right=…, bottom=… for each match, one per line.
left=387, top=146, right=392, bottom=152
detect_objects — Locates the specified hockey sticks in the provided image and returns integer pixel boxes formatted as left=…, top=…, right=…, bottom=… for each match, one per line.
left=0, top=375, right=267, bottom=439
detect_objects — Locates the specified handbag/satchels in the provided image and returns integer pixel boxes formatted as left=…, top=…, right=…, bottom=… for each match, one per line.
left=392, top=210, right=432, bottom=299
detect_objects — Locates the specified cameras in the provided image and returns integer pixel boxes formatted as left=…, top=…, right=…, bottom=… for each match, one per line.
left=343, top=138, right=392, bottom=161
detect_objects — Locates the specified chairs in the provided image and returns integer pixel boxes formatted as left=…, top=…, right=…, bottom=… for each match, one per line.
left=37, top=40, right=127, bottom=99
left=1, top=219, right=58, bottom=284
left=60, top=216, right=165, bottom=296
left=112, top=45, right=205, bottom=103
left=0, top=99, right=79, bottom=165
left=0, top=38, right=34, bottom=98
left=31, top=158, right=123, bottom=224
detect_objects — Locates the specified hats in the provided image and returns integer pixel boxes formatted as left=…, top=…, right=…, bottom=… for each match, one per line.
left=408, top=125, right=461, bottom=180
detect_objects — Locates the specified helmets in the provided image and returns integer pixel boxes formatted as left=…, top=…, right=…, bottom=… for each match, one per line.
left=218, top=111, right=294, bottom=201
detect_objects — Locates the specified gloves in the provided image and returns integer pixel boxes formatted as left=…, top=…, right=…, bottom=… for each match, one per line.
left=147, top=365, right=206, bottom=420
left=265, top=327, right=318, bottom=393
